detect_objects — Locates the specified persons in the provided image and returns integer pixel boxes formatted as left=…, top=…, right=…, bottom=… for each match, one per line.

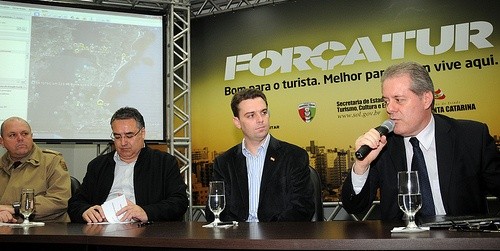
left=0, top=116, right=72, bottom=223
left=205, top=89, right=315, bottom=224
left=341, top=61, right=500, bottom=222
left=67, top=106, right=189, bottom=224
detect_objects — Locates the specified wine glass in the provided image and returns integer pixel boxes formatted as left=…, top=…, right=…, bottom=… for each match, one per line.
left=20, top=188, right=35, bottom=225
left=209, top=181, right=226, bottom=227
left=396, top=170, right=424, bottom=232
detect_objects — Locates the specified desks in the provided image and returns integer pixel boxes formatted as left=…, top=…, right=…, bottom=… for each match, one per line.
left=0, top=222, right=500, bottom=251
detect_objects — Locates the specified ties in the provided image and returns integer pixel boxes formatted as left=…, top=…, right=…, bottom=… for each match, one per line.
left=409, top=137, right=436, bottom=216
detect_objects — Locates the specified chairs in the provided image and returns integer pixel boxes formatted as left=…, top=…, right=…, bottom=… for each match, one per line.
left=309, top=165, right=324, bottom=222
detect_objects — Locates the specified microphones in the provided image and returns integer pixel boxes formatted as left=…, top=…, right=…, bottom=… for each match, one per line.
left=355, top=118, right=396, bottom=160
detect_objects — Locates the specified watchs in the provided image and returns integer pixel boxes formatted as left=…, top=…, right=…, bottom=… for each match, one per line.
left=12, top=201, right=21, bottom=219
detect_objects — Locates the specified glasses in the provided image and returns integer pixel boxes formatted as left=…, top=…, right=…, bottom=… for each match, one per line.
left=110, top=127, right=143, bottom=140
